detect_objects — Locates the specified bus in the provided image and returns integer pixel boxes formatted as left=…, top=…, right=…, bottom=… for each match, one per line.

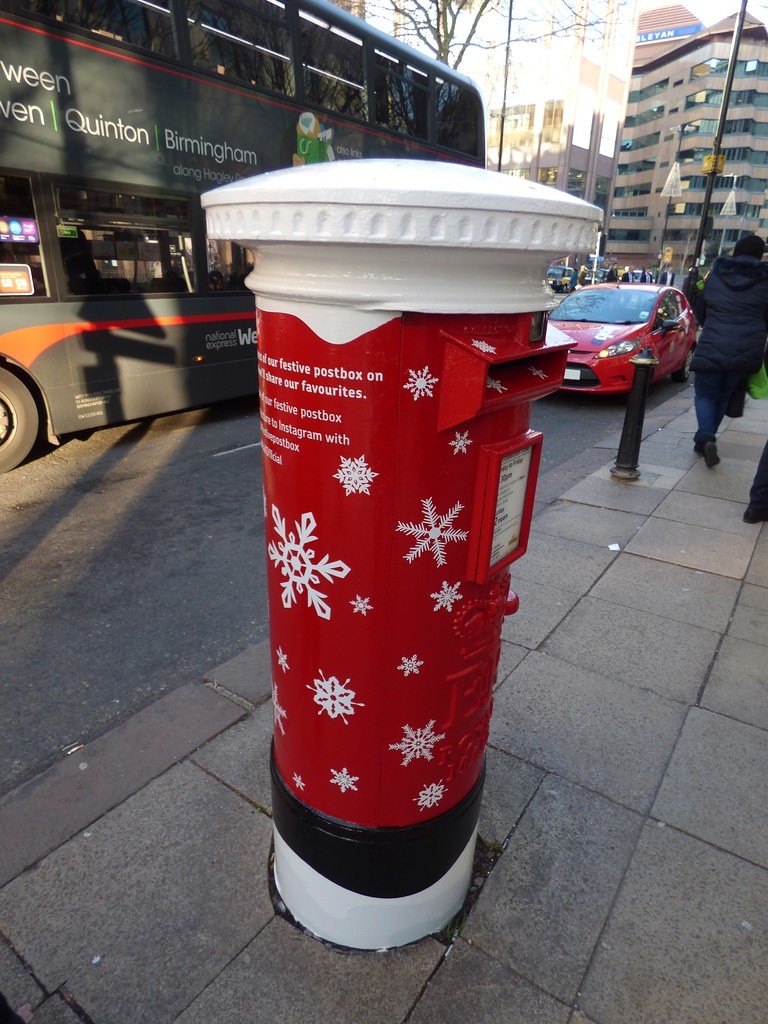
left=0, top=0, right=486, bottom=473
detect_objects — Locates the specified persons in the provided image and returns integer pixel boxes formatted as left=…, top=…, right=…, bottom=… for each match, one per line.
left=682, top=267, right=704, bottom=332
left=577, top=266, right=587, bottom=286
left=659, top=265, right=675, bottom=286
left=622, top=266, right=635, bottom=283
left=743, top=339, right=768, bottom=523
left=689, top=234, right=768, bottom=466
left=606, top=262, right=618, bottom=282
left=640, top=268, right=652, bottom=283
left=568, top=268, right=577, bottom=293
left=208, top=271, right=222, bottom=291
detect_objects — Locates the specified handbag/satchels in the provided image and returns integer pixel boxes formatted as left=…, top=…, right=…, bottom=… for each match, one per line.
left=747, top=361, right=767, bottom=400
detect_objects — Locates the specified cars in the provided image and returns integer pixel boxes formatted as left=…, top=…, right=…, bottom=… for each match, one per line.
left=547, top=280, right=698, bottom=398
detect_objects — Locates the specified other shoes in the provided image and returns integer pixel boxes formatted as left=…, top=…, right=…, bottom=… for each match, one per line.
left=742, top=505, right=768, bottom=524
left=691, top=432, right=720, bottom=468
